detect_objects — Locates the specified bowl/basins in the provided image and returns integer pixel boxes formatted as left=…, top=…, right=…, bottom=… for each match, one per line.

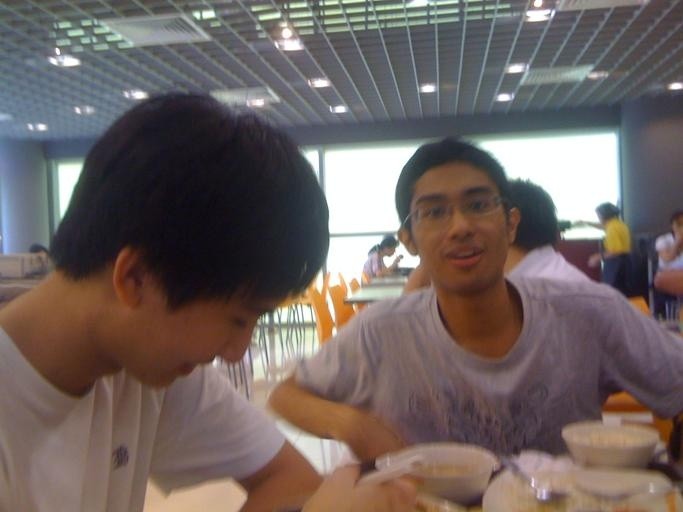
left=560, top=418, right=660, bottom=468
left=374, top=440, right=501, bottom=505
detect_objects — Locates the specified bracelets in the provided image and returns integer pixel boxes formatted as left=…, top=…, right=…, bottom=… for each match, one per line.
left=600, top=253, right=607, bottom=261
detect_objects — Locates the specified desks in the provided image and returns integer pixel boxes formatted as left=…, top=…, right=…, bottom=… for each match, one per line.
left=345, top=273, right=406, bottom=303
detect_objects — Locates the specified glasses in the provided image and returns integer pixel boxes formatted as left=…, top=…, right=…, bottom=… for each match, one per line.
left=400, top=189, right=512, bottom=237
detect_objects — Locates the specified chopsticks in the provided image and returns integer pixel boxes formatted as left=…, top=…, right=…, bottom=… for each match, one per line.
left=356, top=454, right=426, bottom=484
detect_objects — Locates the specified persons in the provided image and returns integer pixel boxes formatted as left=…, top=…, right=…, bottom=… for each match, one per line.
left=653, top=211, right=683, bottom=331
left=268, top=133, right=683, bottom=464
left=0, top=87, right=416, bottom=512
left=404, top=177, right=623, bottom=408
left=586, top=200, right=637, bottom=295
left=360, top=233, right=402, bottom=285
left=27, top=243, right=48, bottom=258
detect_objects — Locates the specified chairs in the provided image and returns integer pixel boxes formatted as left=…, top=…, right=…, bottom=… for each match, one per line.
left=220, top=270, right=377, bottom=399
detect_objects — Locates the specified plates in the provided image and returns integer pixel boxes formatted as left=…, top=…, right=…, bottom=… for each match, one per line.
left=480, top=462, right=676, bottom=512
